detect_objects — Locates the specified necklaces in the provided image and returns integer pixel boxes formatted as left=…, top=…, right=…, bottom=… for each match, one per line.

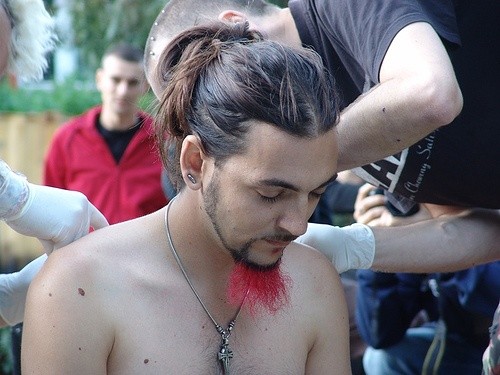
left=165, top=194, right=253, bottom=375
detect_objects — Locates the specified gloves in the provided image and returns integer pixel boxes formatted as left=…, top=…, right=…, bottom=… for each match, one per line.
left=5, top=181, right=110, bottom=255
left=294, top=223, right=375, bottom=274
left=0, top=253, right=48, bottom=327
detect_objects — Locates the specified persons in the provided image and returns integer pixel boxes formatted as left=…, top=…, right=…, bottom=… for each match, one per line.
left=0, top=1, right=499, bottom=374
